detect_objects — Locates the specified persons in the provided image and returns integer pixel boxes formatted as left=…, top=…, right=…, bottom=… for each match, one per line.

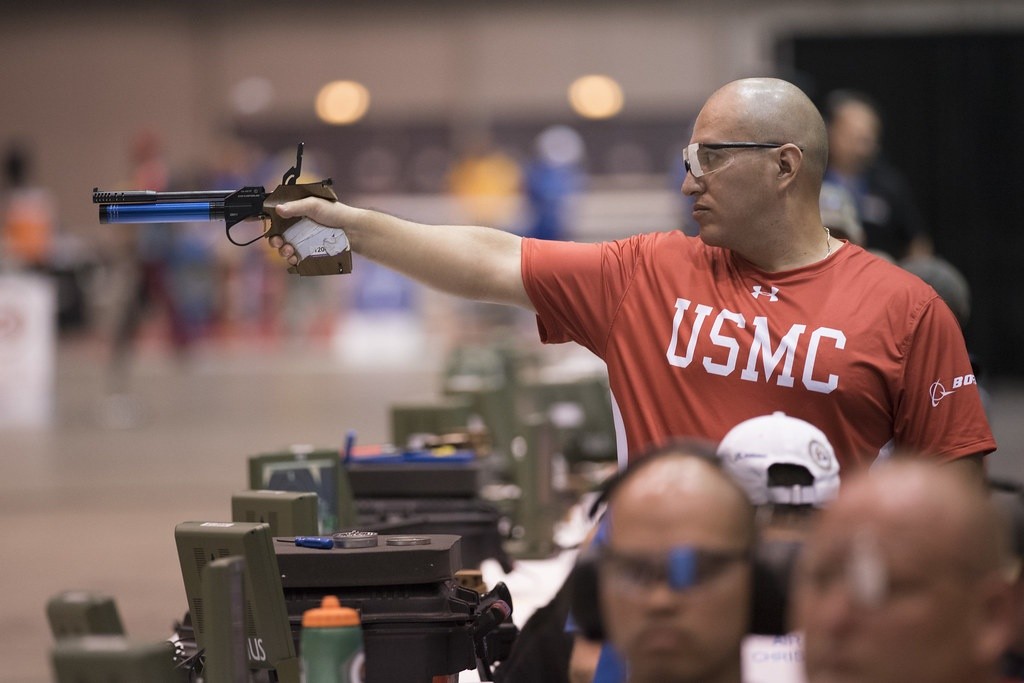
left=819, top=90, right=933, bottom=266
left=518, top=125, right=599, bottom=243
left=495, top=410, right=1024, bottom=682
left=785, top=453, right=1024, bottom=682
left=269, top=77, right=997, bottom=683
left=0, top=136, right=98, bottom=333
left=597, top=453, right=751, bottom=682
left=110, top=122, right=196, bottom=368
left=440, top=107, right=535, bottom=393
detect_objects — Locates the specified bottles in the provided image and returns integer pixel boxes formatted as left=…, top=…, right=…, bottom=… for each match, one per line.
left=300, top=594, right=366, bottom=682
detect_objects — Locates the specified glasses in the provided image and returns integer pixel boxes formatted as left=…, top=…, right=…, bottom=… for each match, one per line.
left=599, top=545, right=750, bottom=590
left=683, top=141, right=804, bottom=178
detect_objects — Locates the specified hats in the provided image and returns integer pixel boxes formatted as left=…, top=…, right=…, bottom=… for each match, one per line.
left=713, top=411, right=840, bottom=507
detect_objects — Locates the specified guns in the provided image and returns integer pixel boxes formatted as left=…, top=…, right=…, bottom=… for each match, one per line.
left=89, top=138, right=357, bottom=277
